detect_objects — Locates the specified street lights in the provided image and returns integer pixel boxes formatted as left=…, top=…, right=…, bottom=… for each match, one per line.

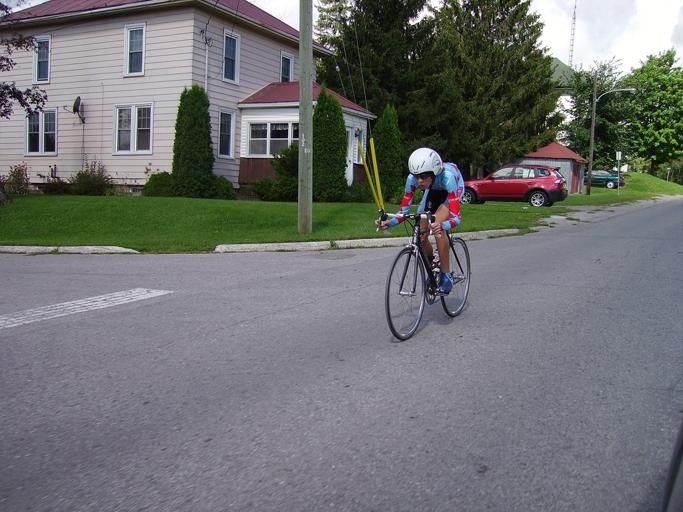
left=586, top=71, right=636, bottom=197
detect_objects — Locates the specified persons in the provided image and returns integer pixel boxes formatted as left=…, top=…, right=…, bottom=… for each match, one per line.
left=374, top=148, right=465, bottom=293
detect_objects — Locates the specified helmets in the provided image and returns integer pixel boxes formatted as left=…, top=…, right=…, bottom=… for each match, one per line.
left=408, top=147, right=443, bottom=176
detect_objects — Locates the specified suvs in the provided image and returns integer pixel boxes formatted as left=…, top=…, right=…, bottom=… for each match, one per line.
left=459, top=163, right=569, bottom=209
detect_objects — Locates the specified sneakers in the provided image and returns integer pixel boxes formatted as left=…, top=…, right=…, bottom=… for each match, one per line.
left=437, top=270, right=454, bottom=294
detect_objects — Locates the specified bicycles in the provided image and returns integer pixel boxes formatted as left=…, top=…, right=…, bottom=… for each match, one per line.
left=375, top=201, right=472, bottom=341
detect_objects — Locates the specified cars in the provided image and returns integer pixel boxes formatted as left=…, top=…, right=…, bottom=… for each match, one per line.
left=584, top=170, right=623, bottom=191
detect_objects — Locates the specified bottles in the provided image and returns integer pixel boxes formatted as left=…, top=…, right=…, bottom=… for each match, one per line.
left=432, top=249, right=442, bottom=272
